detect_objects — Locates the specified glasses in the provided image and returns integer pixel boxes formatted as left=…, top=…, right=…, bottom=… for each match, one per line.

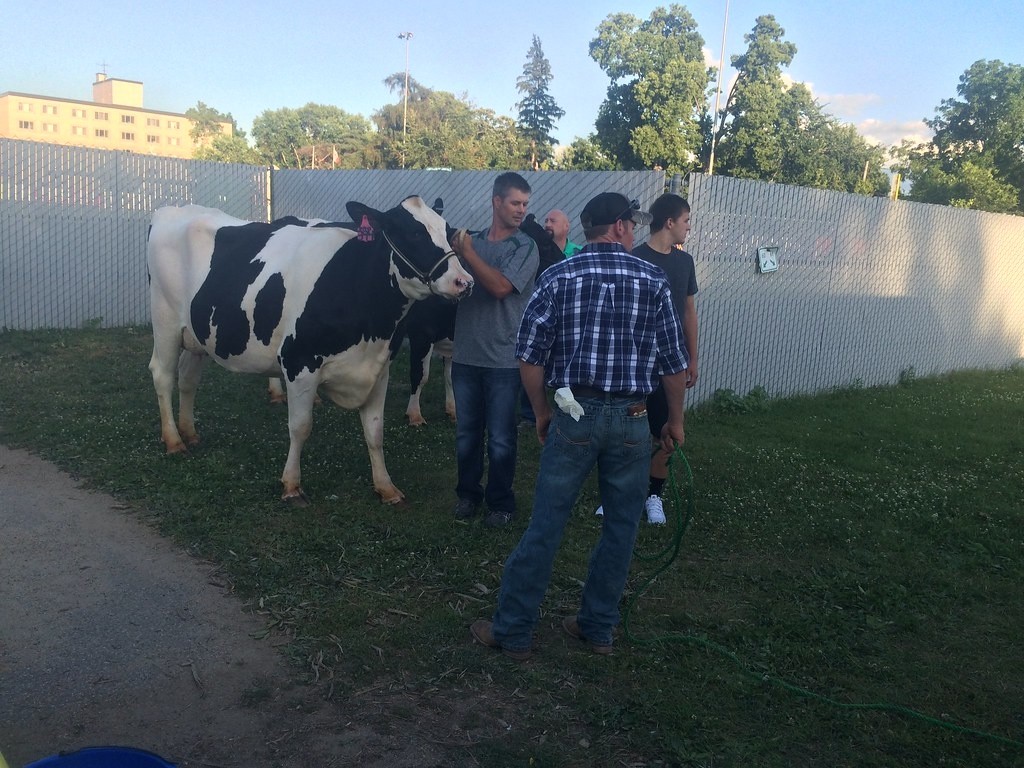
left=616, top=198, right=641, bottom=222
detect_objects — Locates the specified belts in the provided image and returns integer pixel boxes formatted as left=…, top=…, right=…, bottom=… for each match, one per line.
left=573, top=385, right=648, bottom=400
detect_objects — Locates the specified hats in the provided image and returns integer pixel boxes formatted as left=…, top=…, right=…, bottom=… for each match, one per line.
left=580, top=191, right=654, bottom=228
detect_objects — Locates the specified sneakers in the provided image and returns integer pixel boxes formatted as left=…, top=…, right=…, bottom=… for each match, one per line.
left=595, top=505, right=604, bottom=519
left=452, top=500, right=480, bottom=526
left=484, top=511, right=512, bottom=529
left=644, top=494, right=667, bottom=525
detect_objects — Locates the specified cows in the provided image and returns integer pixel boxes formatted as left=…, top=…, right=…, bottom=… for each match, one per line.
left=266, top=211, right=567, bottom=428
left=144, top=194, right=474, bottom=506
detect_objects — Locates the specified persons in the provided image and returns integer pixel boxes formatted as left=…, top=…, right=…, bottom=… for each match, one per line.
left=470, top=192, right=689, bottom=660
left=518, top=211, right=584, bottom=427
left=453, top=173, right=540, bottom=528
left=595, top=194, right=699, bottom=525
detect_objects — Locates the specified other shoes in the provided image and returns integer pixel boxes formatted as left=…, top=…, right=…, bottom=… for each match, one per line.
left=469, top=618, right=532, bottom=661
left=519, top=407, right=537, bottom=425
left=562, top=615, right=614, bottom=654
